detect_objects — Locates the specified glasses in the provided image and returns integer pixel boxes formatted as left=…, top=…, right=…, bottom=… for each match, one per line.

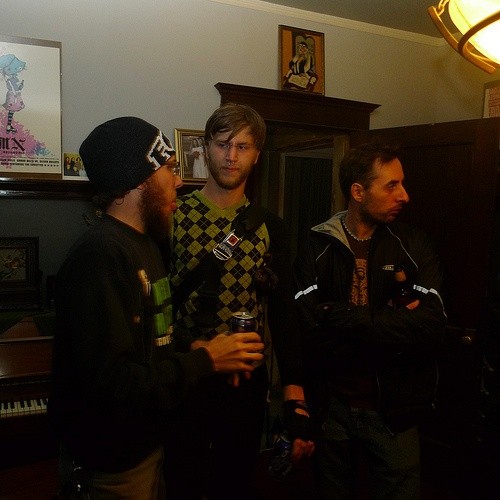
left=164, top=162, right=180, bottom=174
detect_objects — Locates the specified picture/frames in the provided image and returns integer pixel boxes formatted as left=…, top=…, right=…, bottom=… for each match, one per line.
left=278, top=25, right=325, bottom=96
left=0, top=236, right=41, bottom=292
left=61, top=151, right=90, bottom=181
left=173, top=127, right=211, bottom=186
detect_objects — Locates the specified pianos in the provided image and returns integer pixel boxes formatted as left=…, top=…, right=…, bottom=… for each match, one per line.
left=1, top=303, right=56, bottom=424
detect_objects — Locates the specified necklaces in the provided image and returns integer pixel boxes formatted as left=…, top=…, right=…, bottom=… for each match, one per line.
left=342, top=213, right=374, bottom=242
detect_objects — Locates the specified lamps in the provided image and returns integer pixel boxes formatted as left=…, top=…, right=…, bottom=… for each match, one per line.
left=427, top=0, right=500, bottom=74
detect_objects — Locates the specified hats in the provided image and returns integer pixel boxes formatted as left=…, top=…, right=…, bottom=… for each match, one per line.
left=78, top=116, right=176, bottom=196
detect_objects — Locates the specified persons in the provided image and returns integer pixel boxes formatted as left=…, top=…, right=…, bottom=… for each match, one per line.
left=292, top=143, right=446, bottom=500
left=63, top=157, right=82, bottom=176
left=49, top=117, right=264, bottom=500
left=190, top=138, right=210, bottom=180
left=161, top=103, right=316, bottom=500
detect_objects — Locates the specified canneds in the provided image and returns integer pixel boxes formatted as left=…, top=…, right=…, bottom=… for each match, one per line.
left=228, top=312, right=259, bottom=364
left=262, top=435, right=293, bottom=481
